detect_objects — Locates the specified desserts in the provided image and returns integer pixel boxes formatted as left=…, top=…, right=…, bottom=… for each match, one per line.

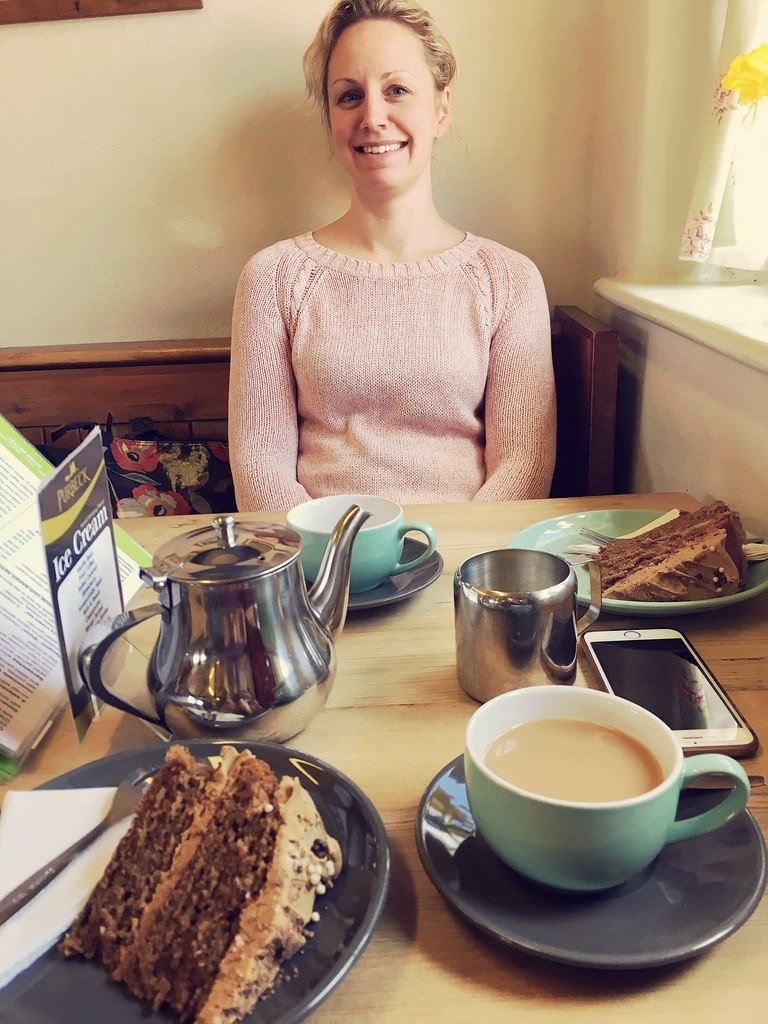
left=59, top=744, right=341, bottom=1024
left=588, top=501, right=747, bottom=600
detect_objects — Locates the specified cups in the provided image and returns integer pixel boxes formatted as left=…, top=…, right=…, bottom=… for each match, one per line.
left=453, top=548, right=603, bottom=704
left=463, top=686, right=751, bottom=891
left=286, top=494, right=438, bottom=594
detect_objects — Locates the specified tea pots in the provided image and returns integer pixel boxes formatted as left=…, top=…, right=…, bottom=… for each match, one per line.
left=77, top=504, right=373, bottom=743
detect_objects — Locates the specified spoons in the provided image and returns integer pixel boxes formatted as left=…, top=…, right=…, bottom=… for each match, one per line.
left=562, top=507, right=681, bottom=553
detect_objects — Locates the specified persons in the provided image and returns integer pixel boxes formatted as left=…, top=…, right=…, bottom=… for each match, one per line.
left=229, top=0, right=559, bottom=512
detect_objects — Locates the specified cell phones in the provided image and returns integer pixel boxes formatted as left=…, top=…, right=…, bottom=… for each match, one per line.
left=579, top=628, right=760, bottom=761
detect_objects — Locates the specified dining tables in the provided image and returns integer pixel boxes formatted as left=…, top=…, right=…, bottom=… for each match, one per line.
left=0, top=484, right=767, bottom=1024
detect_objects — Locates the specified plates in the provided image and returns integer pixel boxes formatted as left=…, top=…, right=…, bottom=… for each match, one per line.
left=505, top=508, right=768, bottom=616
left=0, top=738, right=391, bottom=1024
left=415, top=752, right=768, bottom=968
left=305, top=536, right=444, bottom=611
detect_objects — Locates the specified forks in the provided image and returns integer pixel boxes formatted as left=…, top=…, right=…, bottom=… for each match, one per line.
left=578, top=526, right=763, bottom=547
left=0, top=764, right=164, bottom=926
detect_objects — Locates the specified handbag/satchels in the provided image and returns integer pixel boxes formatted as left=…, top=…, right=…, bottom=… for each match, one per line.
left=50, top=413, right=238, bottom=517
